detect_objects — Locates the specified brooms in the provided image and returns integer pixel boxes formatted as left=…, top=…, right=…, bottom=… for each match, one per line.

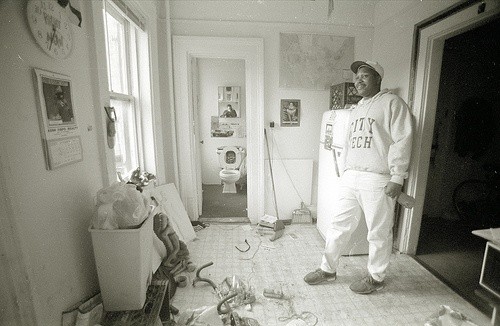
left=262, top=127, right=285, bottom=241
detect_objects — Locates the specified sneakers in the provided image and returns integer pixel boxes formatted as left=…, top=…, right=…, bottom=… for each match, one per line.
left=349, top=272, right=384, bottom=294
left=304, top=268, right=337, bottom=285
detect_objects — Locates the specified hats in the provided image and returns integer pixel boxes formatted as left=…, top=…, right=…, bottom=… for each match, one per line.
left=350, top=59, right=385, bottom=81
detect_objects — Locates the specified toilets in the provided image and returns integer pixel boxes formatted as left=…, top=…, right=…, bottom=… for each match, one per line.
left=216, top=145, right=246, bottom=194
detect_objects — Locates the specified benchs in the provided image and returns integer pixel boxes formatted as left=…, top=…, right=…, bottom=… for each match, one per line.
left=101, top=280, right=169, bottom=326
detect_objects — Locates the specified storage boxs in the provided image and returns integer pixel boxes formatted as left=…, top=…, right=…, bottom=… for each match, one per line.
left=329, top=83, right=363, bottom=110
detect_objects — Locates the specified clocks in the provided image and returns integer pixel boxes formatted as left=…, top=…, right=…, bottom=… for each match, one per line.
left=26, top=0, right=73, bottom=60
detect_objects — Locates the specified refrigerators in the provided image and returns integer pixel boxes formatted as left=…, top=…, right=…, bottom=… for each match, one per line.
left=316, top=106, right=371, bottom=256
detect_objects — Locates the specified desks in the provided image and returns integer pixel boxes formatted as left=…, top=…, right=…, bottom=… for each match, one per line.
left=471, top=228, right=500, bottom=300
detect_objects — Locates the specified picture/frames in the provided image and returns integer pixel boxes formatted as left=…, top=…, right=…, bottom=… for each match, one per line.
left=281, top=99, right=301, bottom=127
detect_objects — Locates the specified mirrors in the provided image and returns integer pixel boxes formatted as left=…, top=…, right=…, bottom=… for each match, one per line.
left=217, top=86, right=241, bottom=118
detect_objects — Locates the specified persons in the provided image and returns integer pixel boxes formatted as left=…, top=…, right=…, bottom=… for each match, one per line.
left=303, top=60, right=413, bottom=294
left=286, top=102, right=296, bottom=125
left=220, top=104, right=237, bottom=118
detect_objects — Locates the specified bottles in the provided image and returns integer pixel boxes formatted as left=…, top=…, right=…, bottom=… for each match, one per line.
left=385, top=186, right=415, bottom=209
